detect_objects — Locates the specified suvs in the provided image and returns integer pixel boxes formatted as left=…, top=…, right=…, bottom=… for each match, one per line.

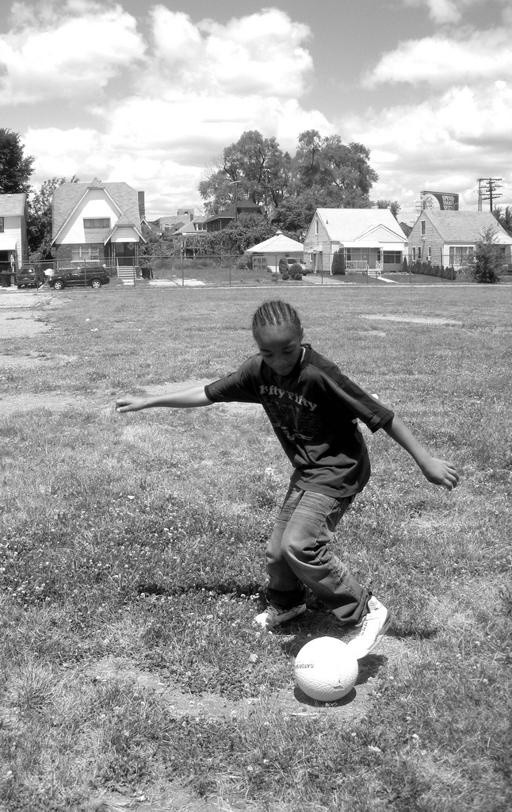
left=48, top=266, right=109, bottom=290
left=15, top=264, right=45, bottom=289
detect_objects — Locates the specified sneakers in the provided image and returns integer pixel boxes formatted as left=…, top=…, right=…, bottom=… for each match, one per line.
left=343, top=596, right=391, bottom=659
left=253, top=603, right=307, bottom=629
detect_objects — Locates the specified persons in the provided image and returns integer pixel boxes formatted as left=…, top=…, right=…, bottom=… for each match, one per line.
left=116, top=300, right=460, bottom=661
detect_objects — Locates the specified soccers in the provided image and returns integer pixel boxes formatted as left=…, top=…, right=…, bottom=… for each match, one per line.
left=293, top=637, right=359, bottom=702
